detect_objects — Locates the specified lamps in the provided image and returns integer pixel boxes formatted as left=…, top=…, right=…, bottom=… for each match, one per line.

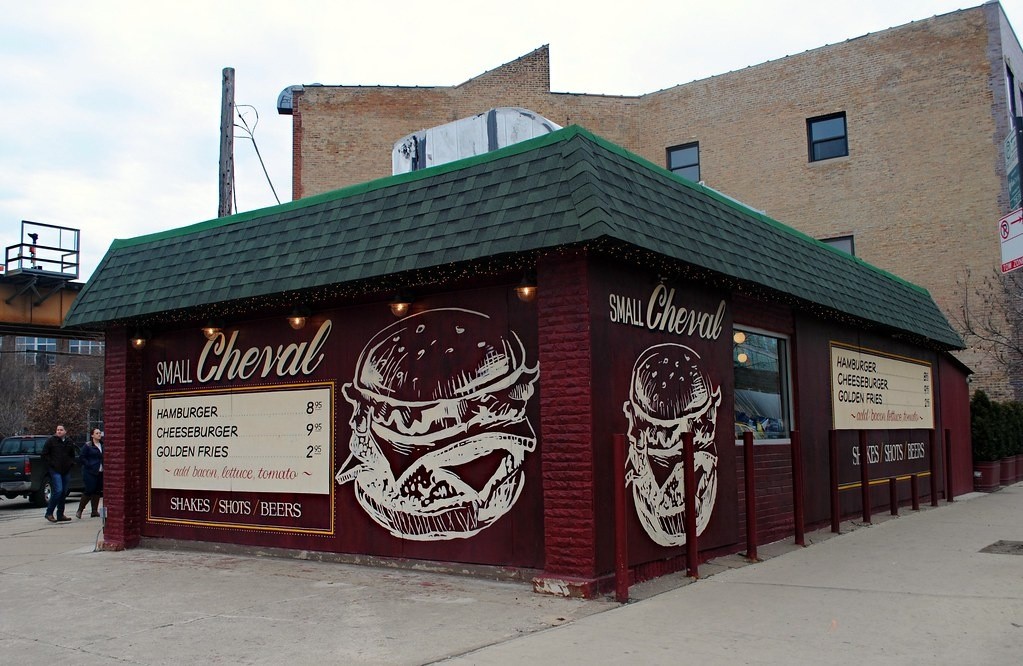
left=200, top=319, right=224, bottom=340
left=129, top=326, right=150, bottom=351
left=387, top=298, right=416, bottom=317
left=512, top=284, right=538, bottom=303
left=285, top=310, right=309, bottom=329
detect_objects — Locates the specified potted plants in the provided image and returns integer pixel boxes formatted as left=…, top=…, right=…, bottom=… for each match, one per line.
left=969, top=389, right=1002, bottom=493
left=995, top=403, right=1015, bottom=485
left=1014, top=400, right=1023, bottom=482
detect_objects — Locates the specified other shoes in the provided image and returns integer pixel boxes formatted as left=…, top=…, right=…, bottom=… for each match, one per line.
left=57, top=515, right=71, bottom=521
left=45, top=514, right=57, bottom=522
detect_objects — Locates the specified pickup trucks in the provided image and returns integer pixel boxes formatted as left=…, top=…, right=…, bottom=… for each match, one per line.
left=0, top=435, right=88, bottom=505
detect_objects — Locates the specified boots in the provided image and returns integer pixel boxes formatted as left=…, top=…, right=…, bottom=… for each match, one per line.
left=91, top=496, right=100, bottom=517
left=76, top=494, right=89, bottom=519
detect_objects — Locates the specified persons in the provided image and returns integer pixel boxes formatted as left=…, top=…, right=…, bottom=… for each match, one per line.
left=76, top=427, right=104, bottom=519
left=40, top=424, right=76, bottom=523
